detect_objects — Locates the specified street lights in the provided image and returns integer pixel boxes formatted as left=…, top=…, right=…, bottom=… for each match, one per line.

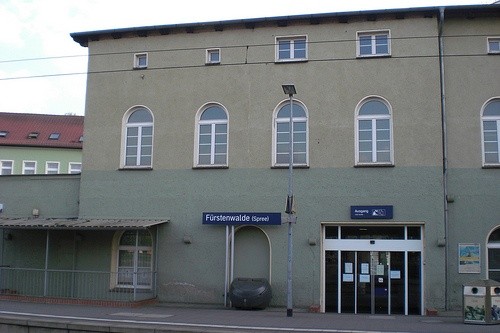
left=279, top=83, right=297, bottom=317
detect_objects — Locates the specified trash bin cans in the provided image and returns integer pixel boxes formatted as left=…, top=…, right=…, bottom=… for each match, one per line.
left=460, top=277, right=500, bottom=324
